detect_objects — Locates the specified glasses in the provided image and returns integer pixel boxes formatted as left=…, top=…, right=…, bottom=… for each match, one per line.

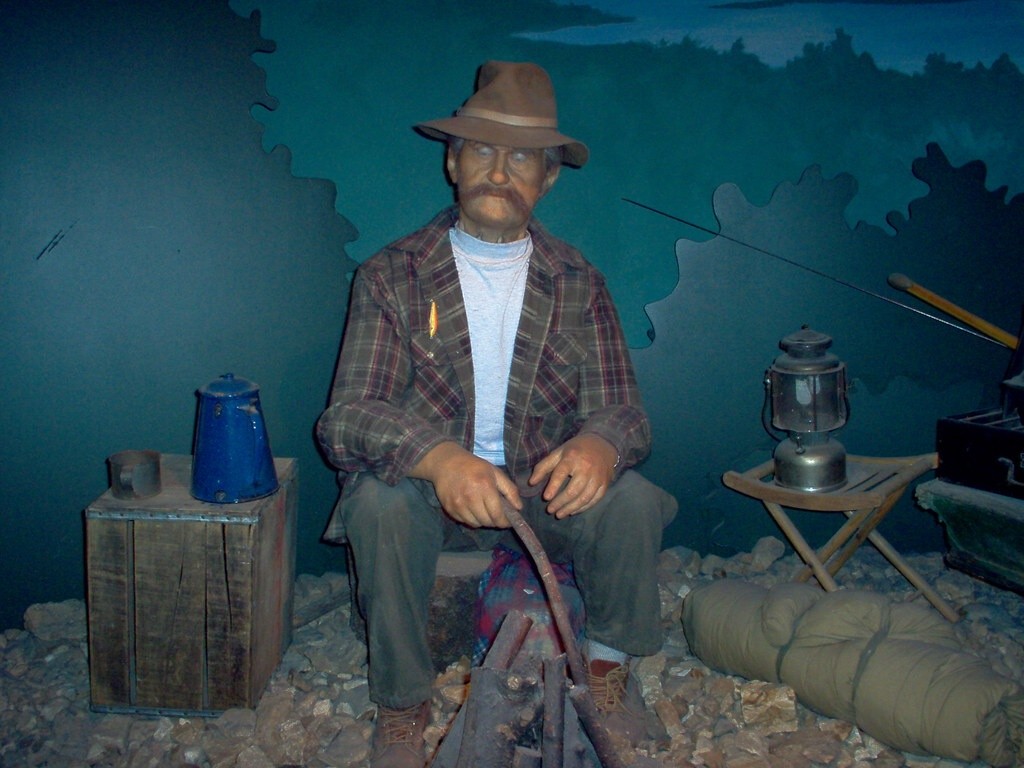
left=463, top=142, right=546, bottom=171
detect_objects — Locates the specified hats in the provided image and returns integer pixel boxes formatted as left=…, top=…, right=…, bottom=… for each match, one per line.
left=416, top=61, right=588, bottom=168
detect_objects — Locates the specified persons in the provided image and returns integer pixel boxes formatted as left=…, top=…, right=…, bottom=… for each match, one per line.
left=315, top=58, right=677, bottom=768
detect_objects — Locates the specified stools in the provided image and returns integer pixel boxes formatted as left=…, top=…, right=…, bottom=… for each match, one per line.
left=726, top=452, right=964, bottom=625
left=324, top=487, right=506, bottom=646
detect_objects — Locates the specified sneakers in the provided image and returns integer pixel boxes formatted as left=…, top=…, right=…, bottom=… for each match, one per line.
left=372, top=699, right=434, bottom=768
left=584, top=657, right=643, bottom=748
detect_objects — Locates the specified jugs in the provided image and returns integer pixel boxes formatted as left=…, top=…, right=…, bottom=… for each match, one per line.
left=190, top=374, right=279, bottom=504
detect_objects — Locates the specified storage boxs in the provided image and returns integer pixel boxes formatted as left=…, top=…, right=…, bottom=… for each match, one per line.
left=80, top=456, right=299, bottom=716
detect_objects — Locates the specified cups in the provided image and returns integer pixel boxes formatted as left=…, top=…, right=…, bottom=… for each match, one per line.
left=108, top=450, right=161, bottom=500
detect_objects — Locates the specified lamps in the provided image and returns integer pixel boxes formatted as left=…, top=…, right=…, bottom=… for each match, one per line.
left=761, top=322, right=854, bottom=494
